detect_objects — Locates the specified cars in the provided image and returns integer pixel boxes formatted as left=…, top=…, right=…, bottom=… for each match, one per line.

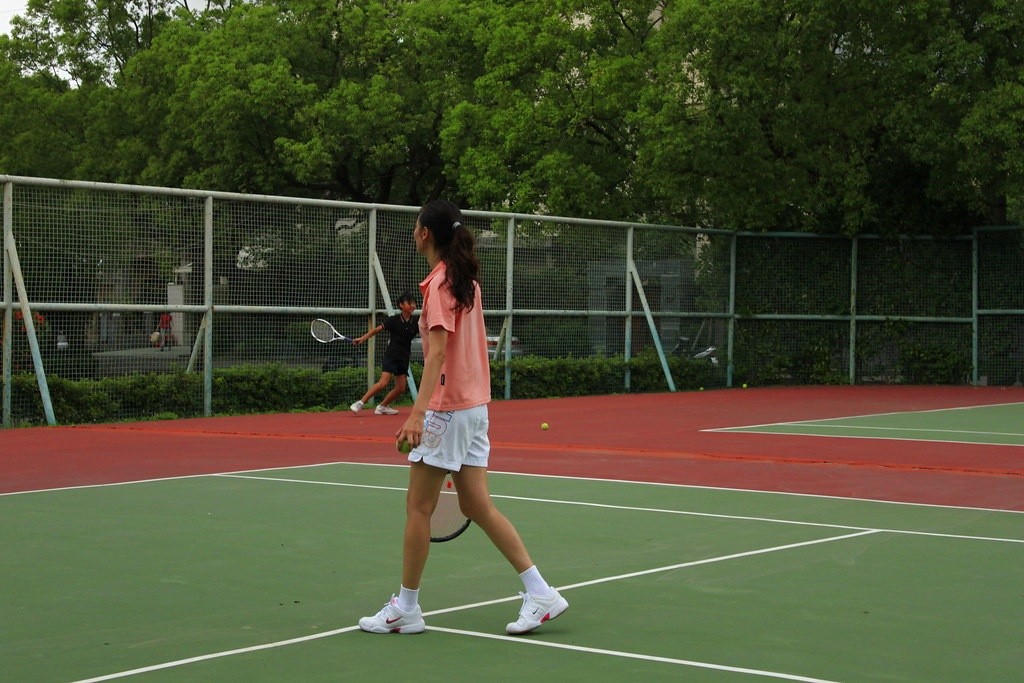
left=410, top=324, right=525, bottom=363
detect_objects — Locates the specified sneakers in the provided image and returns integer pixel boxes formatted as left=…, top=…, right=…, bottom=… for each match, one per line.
left=506, top=586, right=569, bottom=633
left=358, top=592, right=425, bottom=633
left=375, top=404, right=398, bottom=415
left=350, top=401, right=363, bottom=414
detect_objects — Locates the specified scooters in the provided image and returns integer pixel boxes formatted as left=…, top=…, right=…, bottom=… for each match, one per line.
left=670, top=334, right=720, bottom=368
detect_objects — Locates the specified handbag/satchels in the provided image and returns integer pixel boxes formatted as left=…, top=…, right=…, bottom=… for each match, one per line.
left=150, top=332, right=160, bottom=342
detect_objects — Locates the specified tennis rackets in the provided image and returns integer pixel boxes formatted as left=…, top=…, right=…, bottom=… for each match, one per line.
left=311, top=318, right=354, bottom=344
left=430, top=468, right=471, bottom=543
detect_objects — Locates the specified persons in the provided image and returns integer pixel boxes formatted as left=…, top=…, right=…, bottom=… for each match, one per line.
left=358, top=199, right=569, bottom=634
left=156, top=312, right=173, bottom=352
left=350, top=293, right=420, bottom=415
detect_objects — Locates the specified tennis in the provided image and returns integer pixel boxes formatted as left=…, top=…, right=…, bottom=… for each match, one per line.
left=541, top=422, right=549, bottom=430
left=396, top=438, right=413, bottom=454
